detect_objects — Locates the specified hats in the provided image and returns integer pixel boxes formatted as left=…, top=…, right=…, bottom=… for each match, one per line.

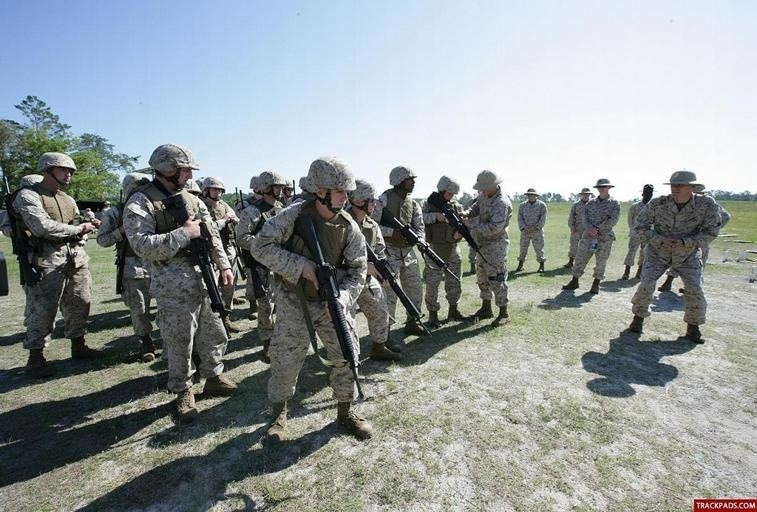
left=86, top=208, right=91, bottom=211
left=579, top=187, right=593, bottom=194
left=663, top=169, right=705, bottom=192
left=593, top=179, right=615, bottom=187
left=104, top=201, right=111, bottom=204
left=524, top=188, right=540, bottom=196
left=473, top=170, right=503, bottom=190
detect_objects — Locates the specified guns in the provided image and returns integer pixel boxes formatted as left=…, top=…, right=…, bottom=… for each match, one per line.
left=2, top=175, right=43, bottom=287
left=160, top=192, right=232, bottom=340
left=380, top=207, right=461, bottom=285
left=293, top=212, right=364, bottom=399
left=114, top=188, right=125, bottom=294
left=427, top=190, right=489, bottom=264
left=239, top=189, right=266, bottom=299
left=365, top=240, right=434, bottom=340
left=234, top=186, right=247, bottom=280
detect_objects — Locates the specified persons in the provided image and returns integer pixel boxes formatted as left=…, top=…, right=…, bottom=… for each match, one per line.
left=281, top=180, right=294, bottom=206
left=564, top=187, right=593, bottom=268
left=199, top=175, right=241, bottom=334
left=96, top=171, right=156, bottom=363
left=233, top=175, right=263, bottom=319
left=0, top=174, right=44, bottom=238
left=622, top=184, right=654, bottom=280
left=516, top=188, right=548, bottom=272
left=468, top=198, right=479, bottom=274
left=418, top=175, right=468, bottom=326
left=459, top=169, right=513, bottom=325
left=102, top=200, right=112, bottom=214
left=236, top=170, right=286, bottom=364
left=629, top=171, right=723, bottom=344
left=371, top=165, right=432, bottom=353
left=344, top=178, right=404, bottom=362
left=121, top=143, right=240, bottom=418
left=85, top=207, right=95, bottom=220
left=657, top=185, right=731, bottom=293
left=250, top=154, right=373, bottom=443
left=11, top=152, right=106, bottom=379
left=562, top=178, right=621, bottom=294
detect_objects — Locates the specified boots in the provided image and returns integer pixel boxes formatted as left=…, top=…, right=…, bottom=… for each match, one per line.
left=142, top=337, right=155, bottom=361
left=591, top=278, right=600, bottom=294
left=563, top=277, right=579, bottom=289
left=428, top=311, right=442, bottom=327
left=71, top=335, right=106, bottom=358
left=448, top=303, right=470, bottom=321
left=629, top=315, right=643, bottom=332
left=491, top=304, right=511, bottom=326
left=233, top=297, right=246, bottom=304
left=385, top=332, right=402, bottom=352
left=407, top=318, right=431, bottom=334
left=337, top=400, right=373, bottom=438
left=471, top=263, right=476, bottom=274
left=177, top=388, right=198, bottom=419
left=565, top=257, right=573, bottom=267
left=538, top=262, right=544, bottom=272
left=203, top=376, right=239, bottom=394
left=263, top=340, right=270, bottom=363
left=24, top=354, right=31, bottom=374
left=262, top=400, right=287, bottom=441
left=636, top=266, right=642, bottom=278
left=373, top=342, right=401, bottom=359
left=248, top=300, right=258, bottom=319
left=469, top=299, right=494, bottom=318
left=31, top=348, right=57, bottom=378
left=517, top=260, right=523, bottom=271
left=679, top=287, right=685, bottom=292
left=687, top=324, right=705, bottom=343
left=225, top=316, right=241, bottom=333
left=658, top=275, right=673, bottom=290
left=622, top=265, right=630, bottom=280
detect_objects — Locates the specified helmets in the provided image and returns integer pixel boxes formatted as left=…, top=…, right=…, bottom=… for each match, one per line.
left=389, top=166, right=417, bottom=186
left=258, top=171, right=286, bottom=190
left=202, top=176, right=225, bottom=193
left=148, top=144, right=200, bottom=170
left=437, top=175, right=460, bottom=194
left=184, top=178, right=202, bottom=195
left=299, top=176, right=307, bottom=190
left=348, top=180, right=379, bottom=201
left=304, top=154, right=357, bottom=193
left=20, top=174, right=44, bottom=186
left=123, top=173, right=149, bottom=202
left=38, top=151, right=77, bottom=177
left=250, top=176, right=259, bottom=189
left=282, top=178, right=293, bottom=189
